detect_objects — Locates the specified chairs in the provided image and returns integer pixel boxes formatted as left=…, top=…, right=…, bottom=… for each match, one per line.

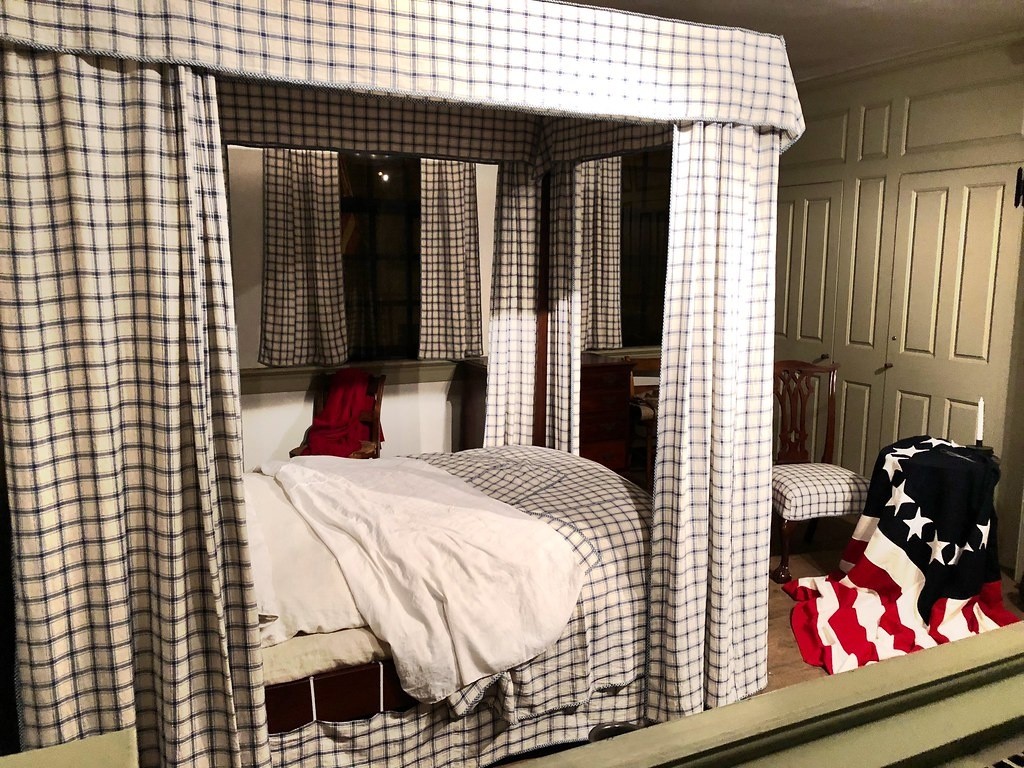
left=242, top=445, right=651, bottom=768
left=289, top=373, right=386, bottom=458
left=770, top=359, right=871, bottom=582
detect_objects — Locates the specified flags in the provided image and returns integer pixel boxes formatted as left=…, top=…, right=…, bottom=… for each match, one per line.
left=781, top=433, right=1019, bottom=677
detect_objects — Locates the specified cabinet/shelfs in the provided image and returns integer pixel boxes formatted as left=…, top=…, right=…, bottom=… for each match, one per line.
left=777, top=29, right=1024, bottom=573
left=461, top=350, right=639, bottom=472
